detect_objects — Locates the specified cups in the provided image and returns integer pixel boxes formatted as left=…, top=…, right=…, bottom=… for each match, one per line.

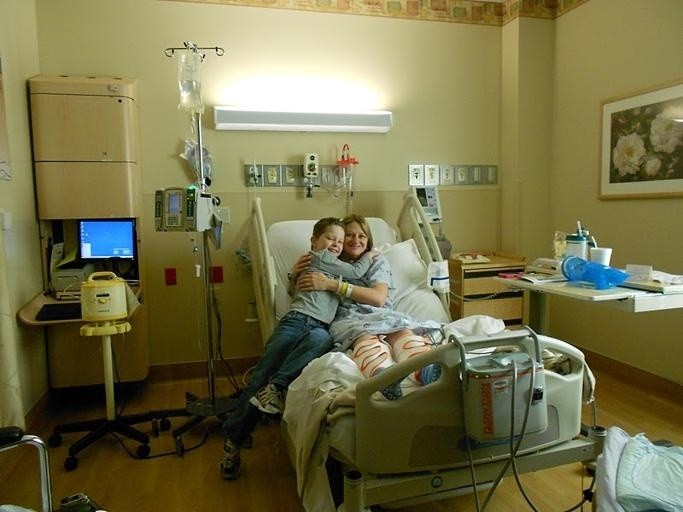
left=565, top=234, right=588, bottom=264
left=590, top=247, right=611, bottom=267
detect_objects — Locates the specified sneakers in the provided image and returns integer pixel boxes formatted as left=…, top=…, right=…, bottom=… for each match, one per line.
left=248, top=384, right=281, bottom=414
left=422, top=362, right=442, bottom=386
left=371, top=366, right=403, bottom=401
left=217, top=433, right=240, bottom=482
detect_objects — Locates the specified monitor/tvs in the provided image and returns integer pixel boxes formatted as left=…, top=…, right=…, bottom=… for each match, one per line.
left=77, top=218, right=138, bottom=262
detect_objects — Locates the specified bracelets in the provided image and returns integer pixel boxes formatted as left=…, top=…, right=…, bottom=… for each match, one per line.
left=336, top=275, right=353, bottom=297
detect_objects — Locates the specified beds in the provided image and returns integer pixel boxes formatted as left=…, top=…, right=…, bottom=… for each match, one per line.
left=245, top=193, right=605, bottom=510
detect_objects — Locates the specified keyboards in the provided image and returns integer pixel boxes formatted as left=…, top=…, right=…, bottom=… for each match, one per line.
left=36, top=302, right=82, bottom=321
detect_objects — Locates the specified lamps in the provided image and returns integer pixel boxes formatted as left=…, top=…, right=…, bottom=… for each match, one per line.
left=214, top=105, right=393, bottom=133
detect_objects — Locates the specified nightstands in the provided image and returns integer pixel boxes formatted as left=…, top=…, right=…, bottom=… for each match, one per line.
left=448, top=252, right=530, bottom=331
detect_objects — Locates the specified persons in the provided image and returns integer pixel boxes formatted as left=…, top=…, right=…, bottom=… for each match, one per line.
left=221, top=216, right=381, bottom=481
left=288, top=214, right=443, bottom=402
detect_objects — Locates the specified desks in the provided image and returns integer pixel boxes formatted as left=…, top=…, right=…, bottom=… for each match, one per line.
left=493, top=260, right=683, bottom=336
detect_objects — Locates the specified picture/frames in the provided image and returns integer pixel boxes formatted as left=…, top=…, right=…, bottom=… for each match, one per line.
left=597, top=78, right=683, bottom=202
